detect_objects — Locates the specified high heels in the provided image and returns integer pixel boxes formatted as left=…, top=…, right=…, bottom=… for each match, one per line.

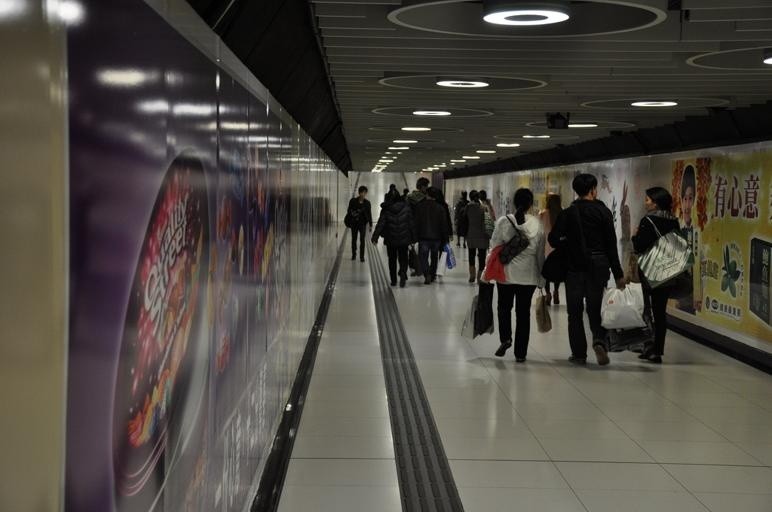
left=455, top=242, right=467, bottom=249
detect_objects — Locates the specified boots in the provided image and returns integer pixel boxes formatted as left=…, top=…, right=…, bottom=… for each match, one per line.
left=468, top=265, right=483, bottom=285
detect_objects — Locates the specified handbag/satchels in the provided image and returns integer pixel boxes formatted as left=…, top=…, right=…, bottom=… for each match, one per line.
left=535, top=287, right=553, bottom=335
left=540, top=203, right=597, bottom=285
left=632, top=215, right=696, bottom=291
left=460, top=278, right=496, bottom=341
left=435, top=250, right=448, bottom=285
left=408, top=246, right=418, bottom=270
left=600, top=281, right=657, bottom=354
left=444, top=237, right=457, bottom=270
left=498, top=215, right=531, bottom=266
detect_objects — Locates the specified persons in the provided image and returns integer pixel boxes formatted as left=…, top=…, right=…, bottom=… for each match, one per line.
left=347, top=185, right=372, bottom=262
left=678, top=164, right=706, bottom=267
left=632, top=186, right=680, bottom=365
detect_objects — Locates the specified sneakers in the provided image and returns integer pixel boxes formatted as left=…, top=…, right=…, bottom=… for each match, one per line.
left=424, top=270, right=435, bottom=284
left=567, top=354, right=587, bottom=366
left=515, top=357, right=526, bottom=364
left=351, top=252, right=365, bottom=263
left=544, top=291, right=560, bottom=306
left=638, top=353, right=663, bottom=364
left=494, top=339, right=513, bottom=358
left=592, top=342, right=610, bottom=365
left=390, top=270, right=405, bottom=288
